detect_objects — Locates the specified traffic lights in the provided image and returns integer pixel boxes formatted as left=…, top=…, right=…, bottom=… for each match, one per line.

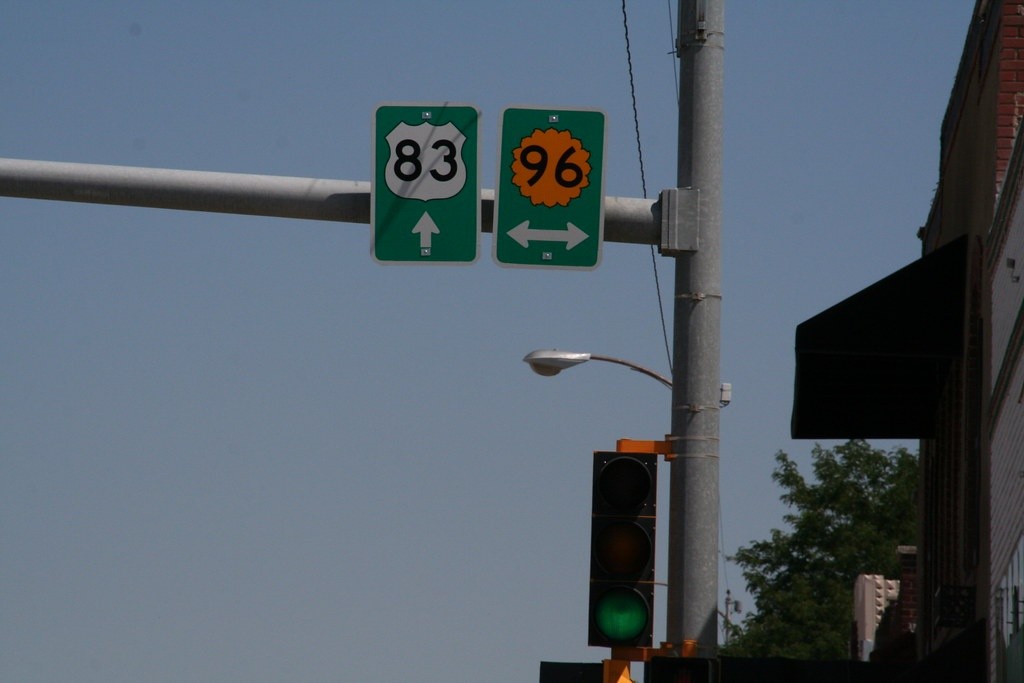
left=587, top=450, right=659, bottom=648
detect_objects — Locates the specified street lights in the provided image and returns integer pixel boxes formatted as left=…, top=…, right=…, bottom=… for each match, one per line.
left=524, top=348, right=731, bottom=683
